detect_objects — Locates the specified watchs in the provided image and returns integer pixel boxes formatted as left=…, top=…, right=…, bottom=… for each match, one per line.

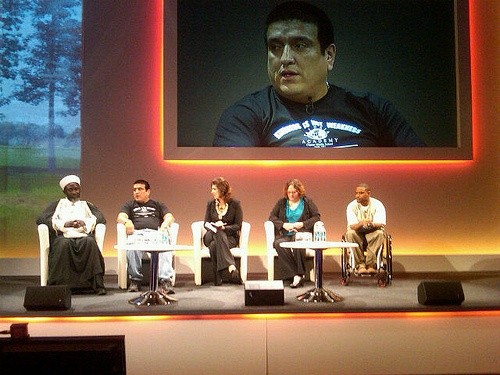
left=367, top=222, right=370, bottom=228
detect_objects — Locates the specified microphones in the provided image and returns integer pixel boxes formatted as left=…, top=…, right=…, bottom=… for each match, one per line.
left=306, top=104, right=314, bottom=114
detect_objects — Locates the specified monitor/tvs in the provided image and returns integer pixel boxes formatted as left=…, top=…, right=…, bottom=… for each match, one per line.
left=0, top=335, right=126, bottom=375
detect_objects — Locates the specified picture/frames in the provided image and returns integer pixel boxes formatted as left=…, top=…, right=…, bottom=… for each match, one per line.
left=162, top=0, right=475, bottom=162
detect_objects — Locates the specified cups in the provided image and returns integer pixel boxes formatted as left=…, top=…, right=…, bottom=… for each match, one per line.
left=295, top=232, right=313, bottom=242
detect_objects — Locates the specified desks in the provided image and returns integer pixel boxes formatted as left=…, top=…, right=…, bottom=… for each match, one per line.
left=115, top=245, right=194, bottom=306
left=280, top=242, right=358, bottom=302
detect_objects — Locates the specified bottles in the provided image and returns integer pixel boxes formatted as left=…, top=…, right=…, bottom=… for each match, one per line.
left=161, top=226, right=168, bottom=245
left=314, top=225, right=326, bottom=241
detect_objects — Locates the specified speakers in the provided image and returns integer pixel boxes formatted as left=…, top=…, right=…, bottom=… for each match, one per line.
left=418, top=281, right=464, bottom=306
left=245, top=280, right=284, bottom=307
left=24, top=285, right=72, bottom=312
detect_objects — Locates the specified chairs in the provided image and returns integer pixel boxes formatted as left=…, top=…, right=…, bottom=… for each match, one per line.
left=191, top=221, right=251, bottom=285
left=38, top=223, right=105, bottom=286
left=265, top=221, right=323, bottom=281
left=117, top=224, right=179, bottom=289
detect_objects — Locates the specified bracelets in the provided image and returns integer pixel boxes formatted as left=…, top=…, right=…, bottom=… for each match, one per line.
left=73, top=221, right=78, bottom=226
left=124, top=220, right=132, bottom=225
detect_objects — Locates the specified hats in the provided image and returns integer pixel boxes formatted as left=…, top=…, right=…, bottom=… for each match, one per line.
left=59, top=175, right=81, bottom=191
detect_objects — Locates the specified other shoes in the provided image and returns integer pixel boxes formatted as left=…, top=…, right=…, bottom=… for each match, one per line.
left=359, top=268, right=367, bottom=274
left=368, top=267, right=376, bottom=274
left=98, top=289, right=107, bottom=296
left=215, top=279, right=223, bottom=286
left=230, top=269, right=239, bottom=281
left=289, top=277, right=305, bottom=288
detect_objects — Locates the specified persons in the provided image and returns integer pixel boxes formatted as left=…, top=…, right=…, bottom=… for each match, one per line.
left=116, top=180, right=176, bottom=294
left=203, top=176, right=243, bottom=286
left=212, top=0, right=426, bottom=147
left=36, top=174, right=105, bottom=295
left=268, top=178, right=321, bottom=288
left=345, top=183, right=387, bottom=276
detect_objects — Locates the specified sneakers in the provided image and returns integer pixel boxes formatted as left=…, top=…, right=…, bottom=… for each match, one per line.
left=129, top=280, right=142, bottom=292
left=161, top=281, right=175, bottom=294
